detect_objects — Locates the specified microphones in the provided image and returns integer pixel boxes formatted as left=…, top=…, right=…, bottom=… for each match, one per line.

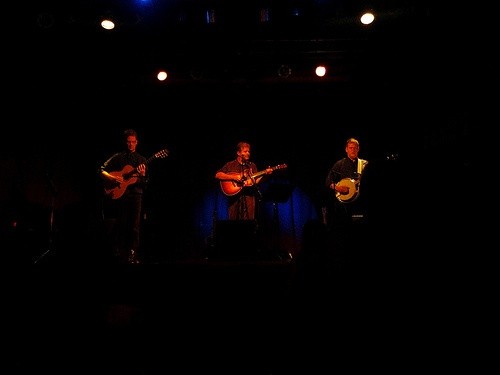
left=331, top=168, right=336, bottom=176
left=242, top=155, right=245, bottom=165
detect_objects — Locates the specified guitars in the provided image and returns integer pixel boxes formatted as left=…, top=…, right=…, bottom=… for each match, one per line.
left=104, top=149, right=169, bottom=199
left=220, top=164, right=287, bottom=196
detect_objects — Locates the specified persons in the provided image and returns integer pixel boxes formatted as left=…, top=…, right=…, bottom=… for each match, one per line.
left=214, top=142, right=273, bottom=220
left=325, top=138, right=369, bottom=194
left=97, top=130, right=148, bottom=262
